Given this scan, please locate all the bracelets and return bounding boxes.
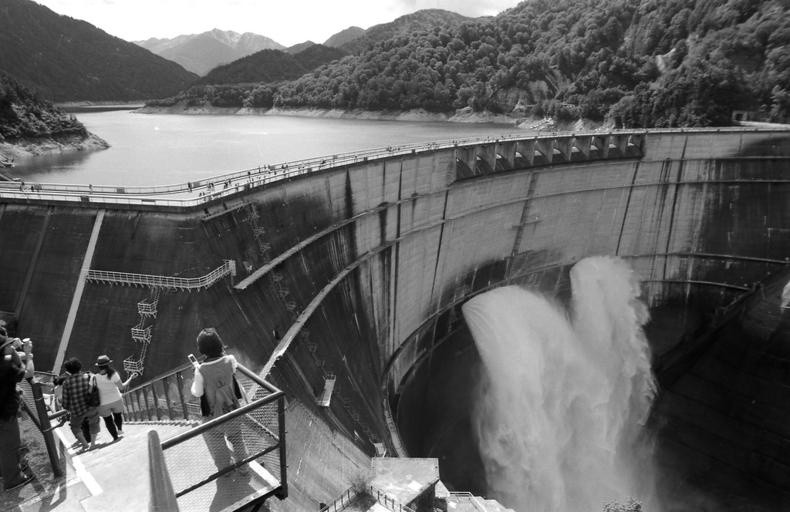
[25,353,35,359]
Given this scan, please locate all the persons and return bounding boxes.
[0,319,38,493]
[87,355,139,442]
[60,356,102,454]
[189,327,252,480]
[52,360,72,425]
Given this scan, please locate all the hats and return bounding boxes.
[94,355,114,367]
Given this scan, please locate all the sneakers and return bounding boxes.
[79,442,102,453]
[117,429,124,434]
[219,464,250,482]
[0,463,36,492]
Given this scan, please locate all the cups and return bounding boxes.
[22,338,30,344]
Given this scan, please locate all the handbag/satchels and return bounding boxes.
[88,375,100,408]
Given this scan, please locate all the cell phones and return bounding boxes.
[188,353,200,368]
